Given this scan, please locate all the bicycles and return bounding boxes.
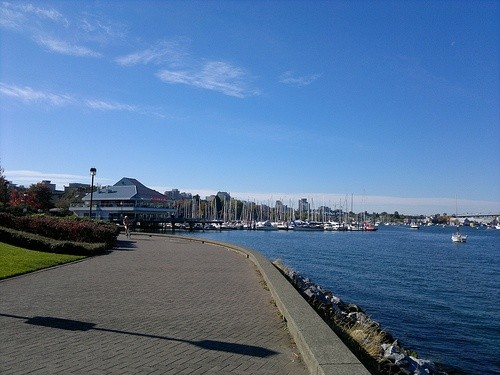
[124,225,131,240]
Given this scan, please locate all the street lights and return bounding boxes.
[90,168,96,218]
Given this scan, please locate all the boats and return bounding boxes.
[452,234,467,243]
[411,223,419,229]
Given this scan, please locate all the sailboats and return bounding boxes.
[158,192,378,232]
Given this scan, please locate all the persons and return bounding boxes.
[122,215,133,237]
